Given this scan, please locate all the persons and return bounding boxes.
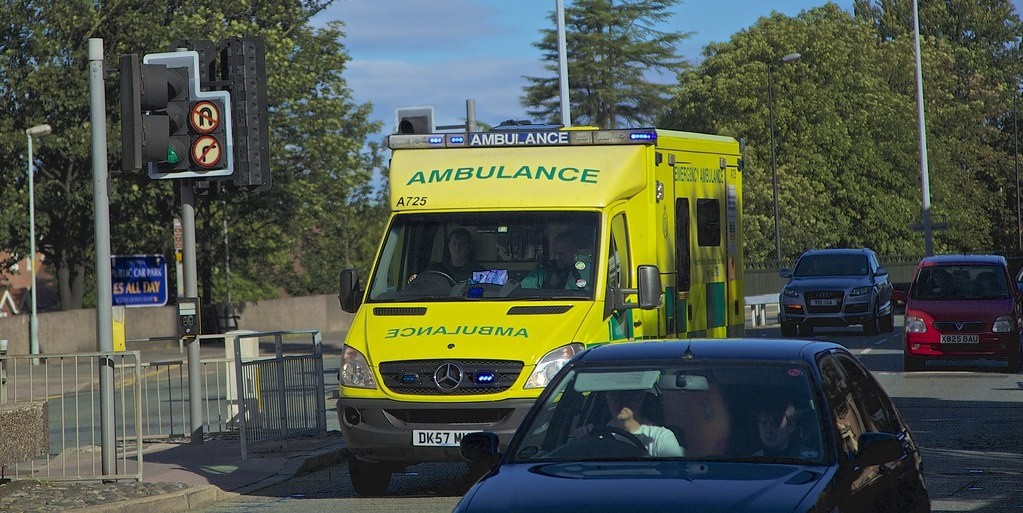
[923,268,962,295]
[407,228,487,286]
[751,400,821,458]
[977,272,1001,298]
[520,232,592,291]
[574,390,687,457]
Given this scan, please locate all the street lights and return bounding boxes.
[25,123,54,365]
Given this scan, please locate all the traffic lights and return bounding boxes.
[396,106,437,134]
[118,53,171,173]
[151,66,189,173]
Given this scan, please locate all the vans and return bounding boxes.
[335,123,748,498]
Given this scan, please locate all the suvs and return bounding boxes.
[777,246,895,338]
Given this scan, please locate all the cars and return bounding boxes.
[889,255,1023,373]
[449,335,933,513]
